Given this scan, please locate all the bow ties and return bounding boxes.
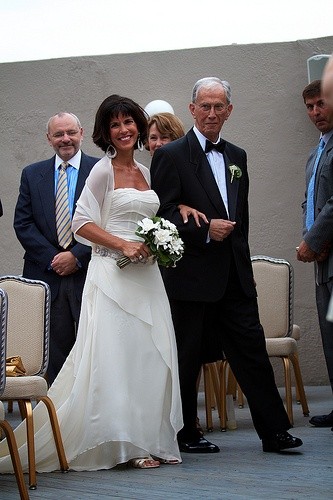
[205,139,225,153]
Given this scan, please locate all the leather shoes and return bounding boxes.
[262,430,303,452]
[176,429,221,453]
[309,410,333,427]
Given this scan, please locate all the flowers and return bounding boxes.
[229,164,243,184]
[113,216,185,270]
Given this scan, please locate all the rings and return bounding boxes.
[138,255,143,261]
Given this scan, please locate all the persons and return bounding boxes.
[296,54,333,433]
[143,109,187,159]
[12,112,104,390]
[149,76,304,455]
[0,94,187,474]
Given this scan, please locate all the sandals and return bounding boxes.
[128,453,181,468]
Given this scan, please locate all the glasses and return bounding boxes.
[191,99,229,113]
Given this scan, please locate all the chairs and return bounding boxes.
[194,254,311,432]
[0,274,70,499]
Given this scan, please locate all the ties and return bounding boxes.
[54,162,73,249]
[306,138,326,231]
